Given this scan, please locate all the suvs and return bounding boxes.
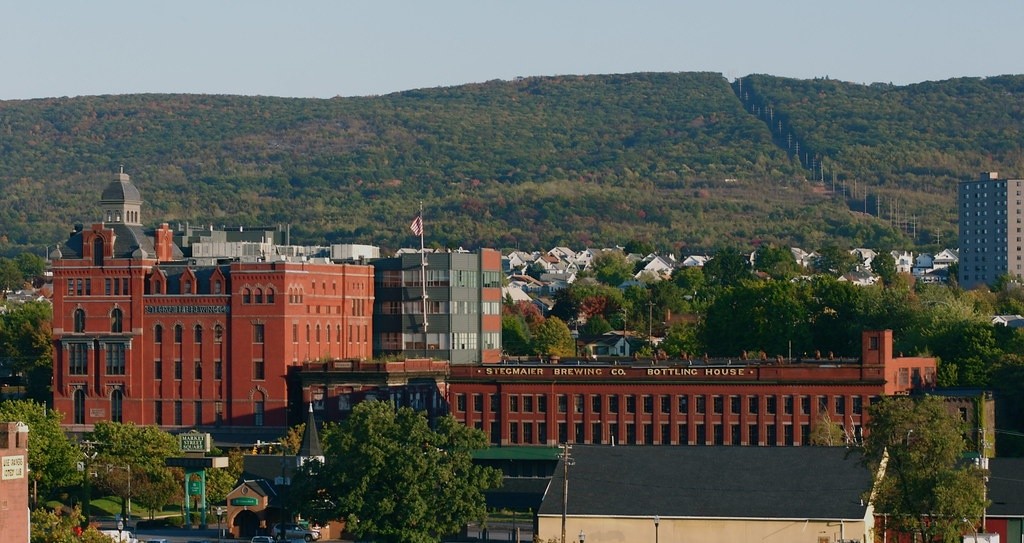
[272,522,322,542]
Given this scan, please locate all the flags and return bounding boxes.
[409,209,424,238]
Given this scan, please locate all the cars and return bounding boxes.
[251,536,275,543]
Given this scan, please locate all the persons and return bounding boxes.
[829,351,833,360]
[751,353,757,360]
[294,512,300,526]
[537,350,541,360]
[758,348,767,362]
[651,348,669,366]
[633,352,638,361]
[585,352,597,362]
[815,349,820,360]
[742,350,747,359]
[775,353,784,365]
[704,352,713,364]
[803,351,807,358]
[501,351,509,364]
[766,362,772,366]
[679,349,696,364]
